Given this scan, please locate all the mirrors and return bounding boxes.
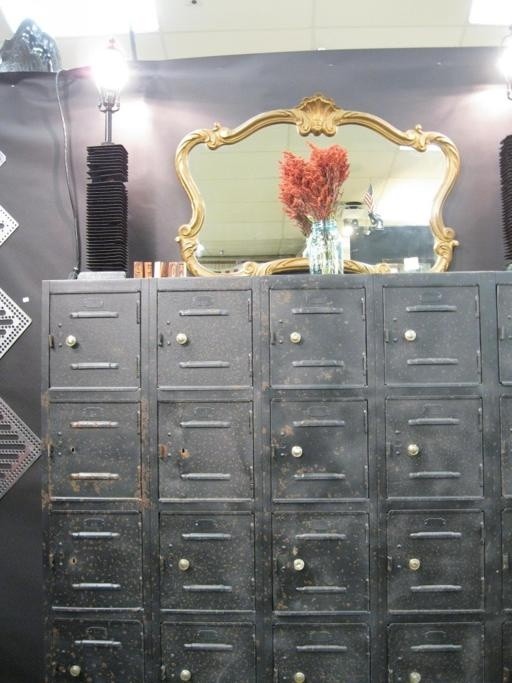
[174,93,461,278]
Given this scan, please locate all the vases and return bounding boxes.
[306,222,344,273]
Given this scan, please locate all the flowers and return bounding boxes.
[278,140,350,273]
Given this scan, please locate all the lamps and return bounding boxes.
[88,38,129,146]
[497,24,512,103]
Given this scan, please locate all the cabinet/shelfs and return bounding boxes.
[40,271,512,683]
